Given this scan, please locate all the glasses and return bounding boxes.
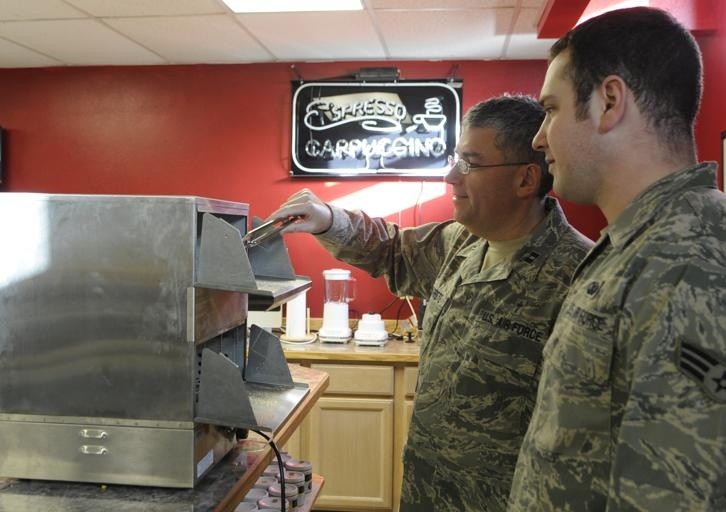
[446,155,544,174]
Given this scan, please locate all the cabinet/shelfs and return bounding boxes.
[303,363,418,509]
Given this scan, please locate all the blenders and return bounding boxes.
[318,268,358,344]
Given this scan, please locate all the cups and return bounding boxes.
[287,292,307,341]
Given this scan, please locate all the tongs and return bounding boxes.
[241,216,304,250]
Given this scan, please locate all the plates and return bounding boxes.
[279,333,318,345]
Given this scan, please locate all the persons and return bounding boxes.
[264,92,597,512]
[506,5,726,512]
